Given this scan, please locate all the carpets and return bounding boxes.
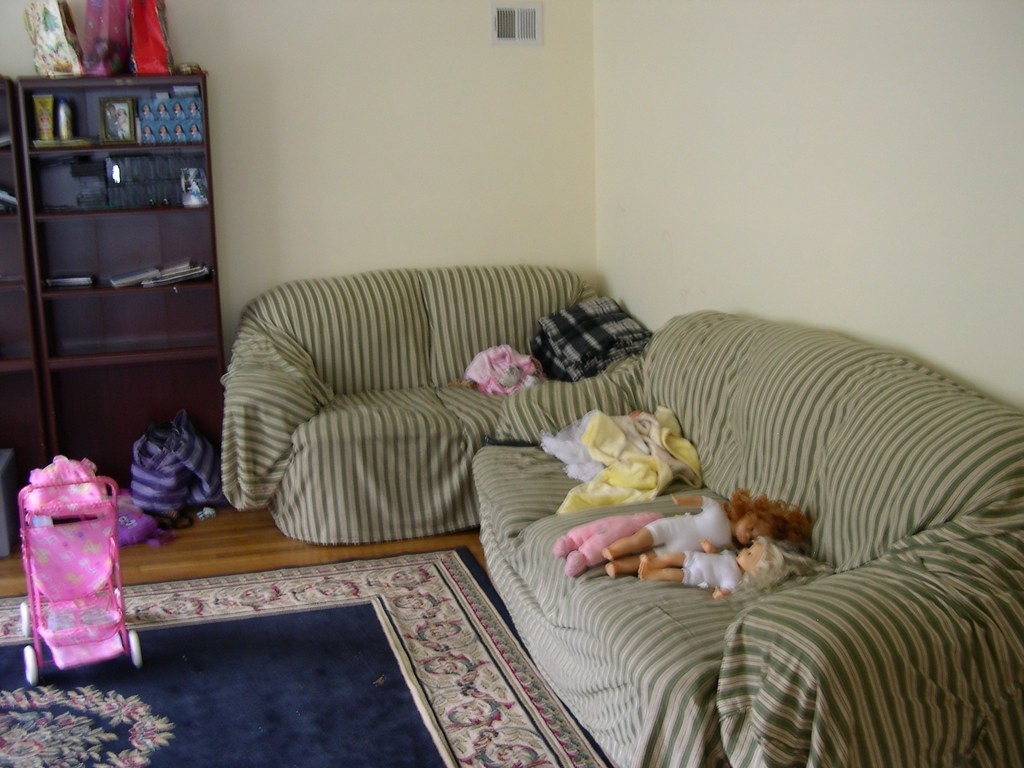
[0,545,617,768]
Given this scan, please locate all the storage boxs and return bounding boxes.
[155,153,205,181]
[69,160,110,208]
[108,183,157,208]
[155,178,183,206]
[104,154,154,184]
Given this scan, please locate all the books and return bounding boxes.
[110,256,210,288]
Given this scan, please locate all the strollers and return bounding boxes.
[17,454,144,687]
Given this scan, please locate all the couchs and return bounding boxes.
[470,310,1024,768]
[219,265,647,548]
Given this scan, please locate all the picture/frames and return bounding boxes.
[98,94,136,145]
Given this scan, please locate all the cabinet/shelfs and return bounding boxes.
[15,73,230,510]
[0,77,50,542]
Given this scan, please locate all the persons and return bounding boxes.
[554,490,819,601]
[104,101,202,196]
